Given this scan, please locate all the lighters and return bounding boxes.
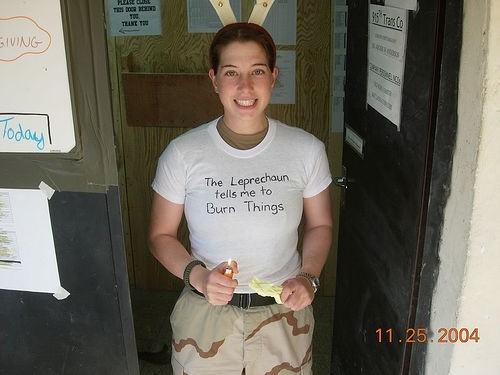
[221,257,234,281]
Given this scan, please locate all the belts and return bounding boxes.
[192,290,277,308]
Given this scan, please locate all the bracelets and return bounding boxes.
[183,259,206,290]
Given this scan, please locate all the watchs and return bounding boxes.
[295,271,321,294]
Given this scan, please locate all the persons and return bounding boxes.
[145,23,333,375]
